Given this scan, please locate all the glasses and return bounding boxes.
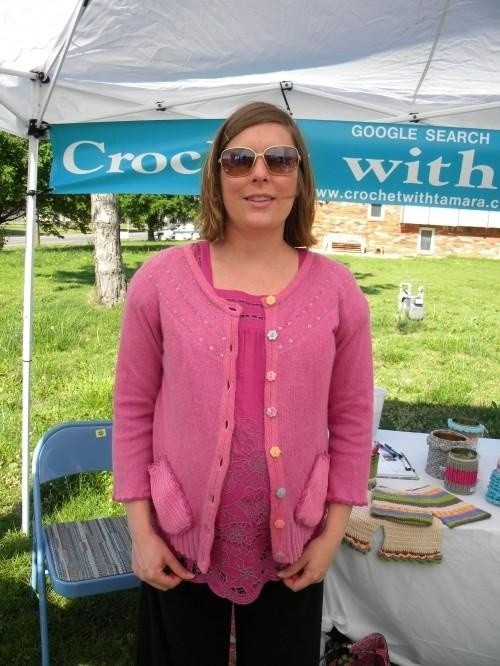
[217,146,301,176]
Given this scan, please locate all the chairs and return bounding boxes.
[30,419,141,665]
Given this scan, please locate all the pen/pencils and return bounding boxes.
[376,440,413,471]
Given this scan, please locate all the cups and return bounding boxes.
[449,418,478,453]
[427,432,466,482]
[442,450,475,495]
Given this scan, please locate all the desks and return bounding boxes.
[322,429,500,666]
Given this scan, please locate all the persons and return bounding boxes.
[112,102,374,666]
